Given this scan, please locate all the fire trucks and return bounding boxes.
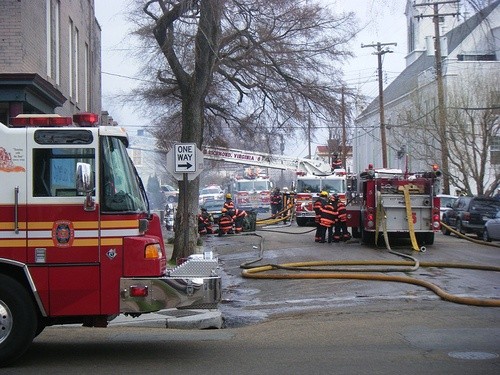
[346,165,440,247]
[123,135,356,227]
[229,168,271,213]
[1,106,223,366]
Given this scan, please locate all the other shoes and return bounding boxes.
[227,231,234,234]
[315,237,348,243]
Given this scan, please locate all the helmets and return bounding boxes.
[329,196,335,201]
[320,191,327,196]
[333,152,338,156]
[334,194,339,200]
[222,208,227,212]
[226,194,232,198]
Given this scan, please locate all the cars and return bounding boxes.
[436,195,462,221]
[439,196,500,239]
[199,185,225,206]
[160,185,180,202]
[481,210,500,241]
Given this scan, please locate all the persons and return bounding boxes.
[272,187,282,223]
[198,208,214,234]
[224,194,235,212]
[495,190,500,201]
[217,208,248,234]
[456,190,468,197]
[331,194,350,241]
[320,196,339,243]
[313,191,329,242]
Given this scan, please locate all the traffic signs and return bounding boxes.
[174,143,197,173]
